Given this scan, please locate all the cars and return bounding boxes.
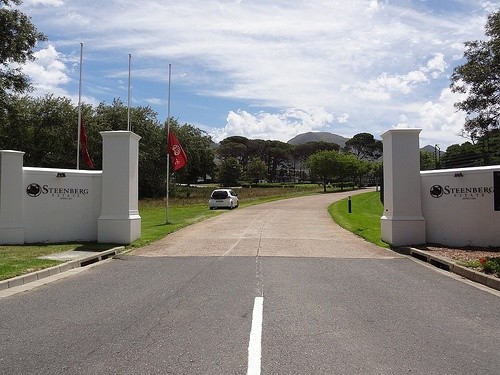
[209,189,239,209]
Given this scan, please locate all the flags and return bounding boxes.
[80,115,95,169]
[165,129,188,172]
